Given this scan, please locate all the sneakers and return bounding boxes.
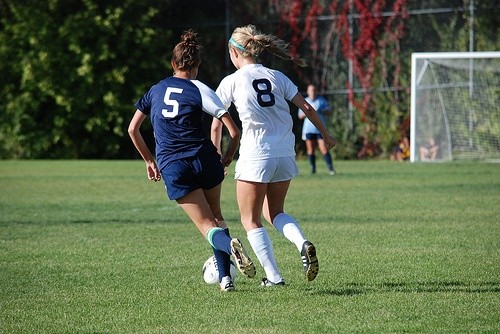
[259,277,285,287]
[229,238,256,278]
[301,241,319,281]
[219,276,235,292]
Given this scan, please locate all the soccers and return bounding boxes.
[202,255,237,284]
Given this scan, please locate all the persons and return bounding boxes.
[210,25,337,288]
[297,84,336,175]
[348,123,451,164]
[128,33,256,292]
